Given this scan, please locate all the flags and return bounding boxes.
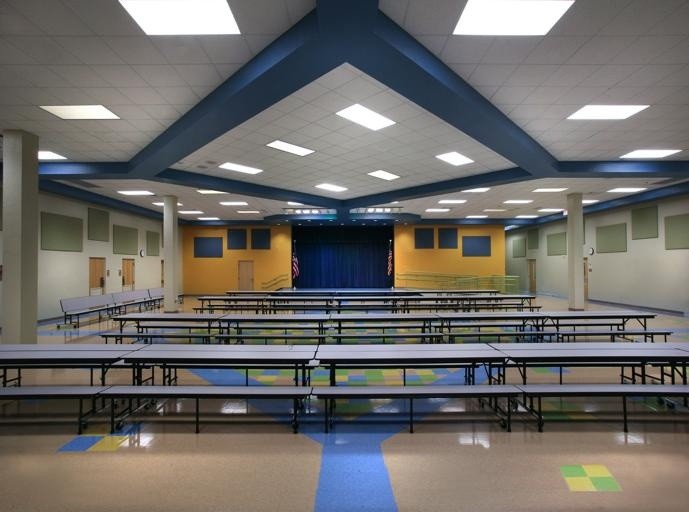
[292,241,300,280]
[386,240,393,277]
